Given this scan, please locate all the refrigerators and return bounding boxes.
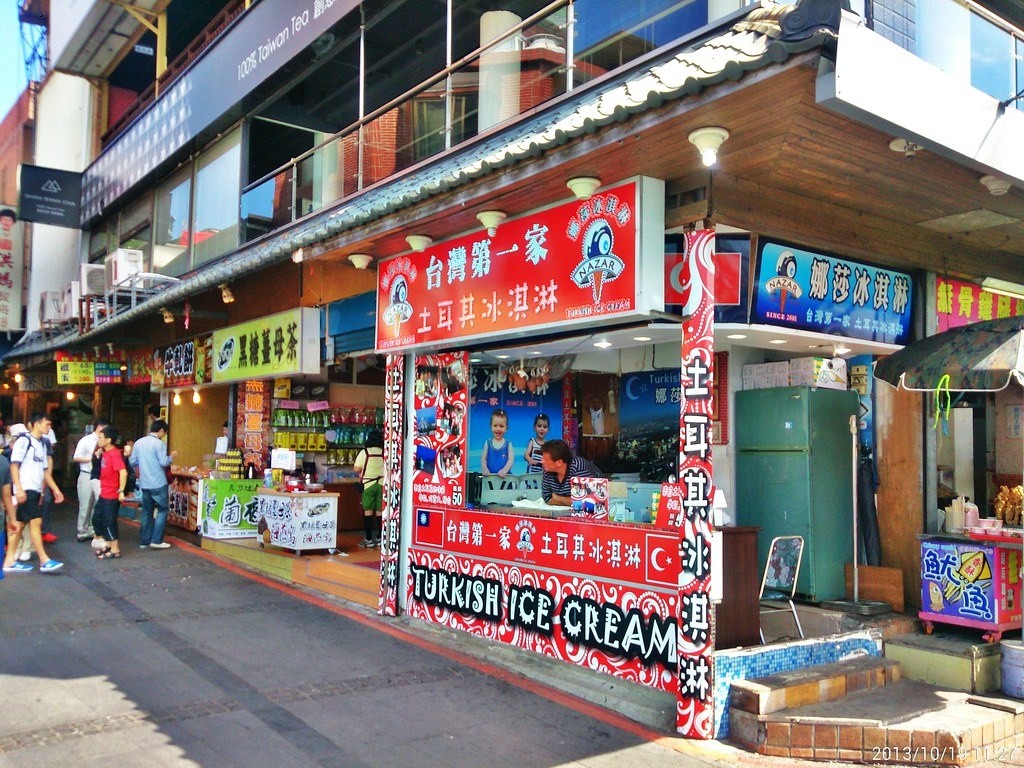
[734,388,860,602]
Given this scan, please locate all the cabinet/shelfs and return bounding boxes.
[269,421,384,476]
[323,482,364,531]
[257,488,340,555]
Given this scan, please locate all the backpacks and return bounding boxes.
[1,432,31,469]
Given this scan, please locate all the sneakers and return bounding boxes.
[375,537,381,547]
[140,544,149,549]
[14,551,33,561]
[150,541,172,549]
[358,538,375,548]
[39,559,64,573]
[1,561,35,573]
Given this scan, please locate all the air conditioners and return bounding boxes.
[40,249,144,323]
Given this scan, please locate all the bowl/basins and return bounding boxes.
[305,483,324,492]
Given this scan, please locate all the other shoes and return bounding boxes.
[90,539,106,549]
[77,529,95,542]
[41,532,58,542]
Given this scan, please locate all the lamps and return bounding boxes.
[819,344,851,356]
[160,307,174,323]
[218,284,234,303]
[80,342,115,362]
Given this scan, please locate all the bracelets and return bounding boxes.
[117,489,123,492]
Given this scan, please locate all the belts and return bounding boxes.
[80,470,92,474]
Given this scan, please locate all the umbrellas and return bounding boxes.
[871,314,1024,392]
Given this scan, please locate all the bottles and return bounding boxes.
[216,448,244,480]
[247,462,255,479]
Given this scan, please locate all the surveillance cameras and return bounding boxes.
[906,149,915,161]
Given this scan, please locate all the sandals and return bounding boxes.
[95,546,111,556]
[97,551,120,559]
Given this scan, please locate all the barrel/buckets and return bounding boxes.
[1000,639,1024,699]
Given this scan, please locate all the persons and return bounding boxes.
[353,429,384,548]
[524,413,550,489]
[129,420,178,548]
[90,425,128,560]
[0,208,17,231]
[0,412,65,572]
[73,418,110,542]
[481,408,514,479]
[540,438,605,507]
[149,404,160,422]
[222,421,228,437]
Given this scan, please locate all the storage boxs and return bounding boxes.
[742,356,848,391]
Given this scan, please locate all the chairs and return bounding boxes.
[480,472,543,506]
[760,536,804,645]
